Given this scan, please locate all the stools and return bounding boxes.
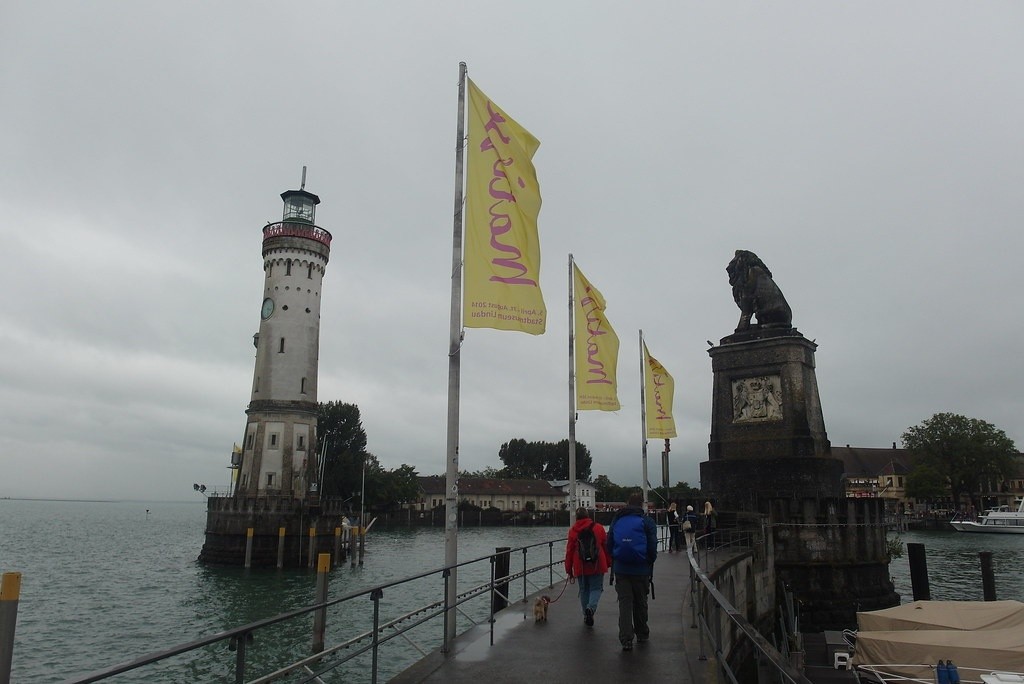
[834,653,849,669]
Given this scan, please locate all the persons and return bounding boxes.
[682,505,698,553]
[605,492,658,652]
[666,502,681,553]
[704,501,719,550]
[563,507,612,627]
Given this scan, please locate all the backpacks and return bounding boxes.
[578,522,599,564]
[610,514,655,600]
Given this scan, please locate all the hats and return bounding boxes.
[687,505,693,511]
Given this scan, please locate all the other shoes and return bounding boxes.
[669,550,672,553]
[623,640,634,650]
[637,638,649,642]
[584,607,594,626]
[676,549,681,552]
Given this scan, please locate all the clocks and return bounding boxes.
[261,298,274,319]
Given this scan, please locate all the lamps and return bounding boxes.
[194,484,200,491]
[200,485,206,492]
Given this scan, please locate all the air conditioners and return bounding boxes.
[231,452,240,465]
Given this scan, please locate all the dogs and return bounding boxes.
[533,595,551,625]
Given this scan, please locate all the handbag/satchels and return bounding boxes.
[683,514,691,530]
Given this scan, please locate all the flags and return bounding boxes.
[462,73,548,336]
[643,339,678,439]
[574,258,622,412]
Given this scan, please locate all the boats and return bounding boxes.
[949,498,1024,534]
[851,598,1024,683]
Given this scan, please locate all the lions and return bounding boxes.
[726,249,792,333]
